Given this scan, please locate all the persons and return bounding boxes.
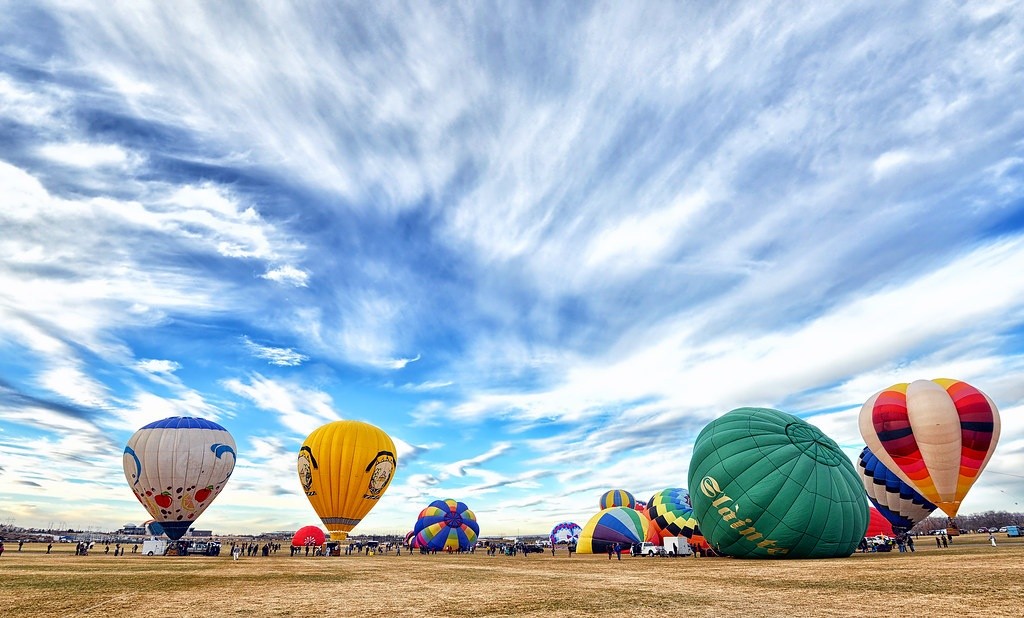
[989,533,997,547]
[673,543,678,557]
[206,544,220,556]
[936,537,942,548]
[606,542,621,560]
[75,541,84,555]
[948,533,952,543]
[941,534,948,548]
[479,540,529,557]
[916,532,918,539]
[567,543,572,558]
[290,545,332,557]
[163,542,193,556]
[690,543,703,558]
[101,540,104,545]
[46,544,52,554]
[262,541,281,556]
[0,540,4,557]
[104,546,109,554]
[114,543,124,556]
[859,534,916,553]
[419,543,477,555]
[18,540,23,551]
[345,540,414,556]
[632,542,638,557]
[105,540,110,545]
[87,540,90,549]
[132,545,138,553]
[227,541,259,560]
[336,541,341,549]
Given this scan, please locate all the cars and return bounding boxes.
[187,540,222,555]
[926,527,947,535]
[977,524,1024,538]
[315,546,324,556]
[859,534,897,549]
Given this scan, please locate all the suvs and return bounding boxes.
[522,544,545,554]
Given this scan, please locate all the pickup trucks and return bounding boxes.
[629,541,665,558]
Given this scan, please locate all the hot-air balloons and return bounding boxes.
[291,525,326,546]
[598,489,636,511]
[403,531,419,549]
[413,498,480,552]
[636,500,646,513]
[643,487,711,557]
[121,415,239,556]
[857,377,1002,537]
[297,420,398,556]
[855,446,938,545]
[548,521,583,545]
[575,507,659,554]
[687,407,871,559]
[864,505,897,539]
